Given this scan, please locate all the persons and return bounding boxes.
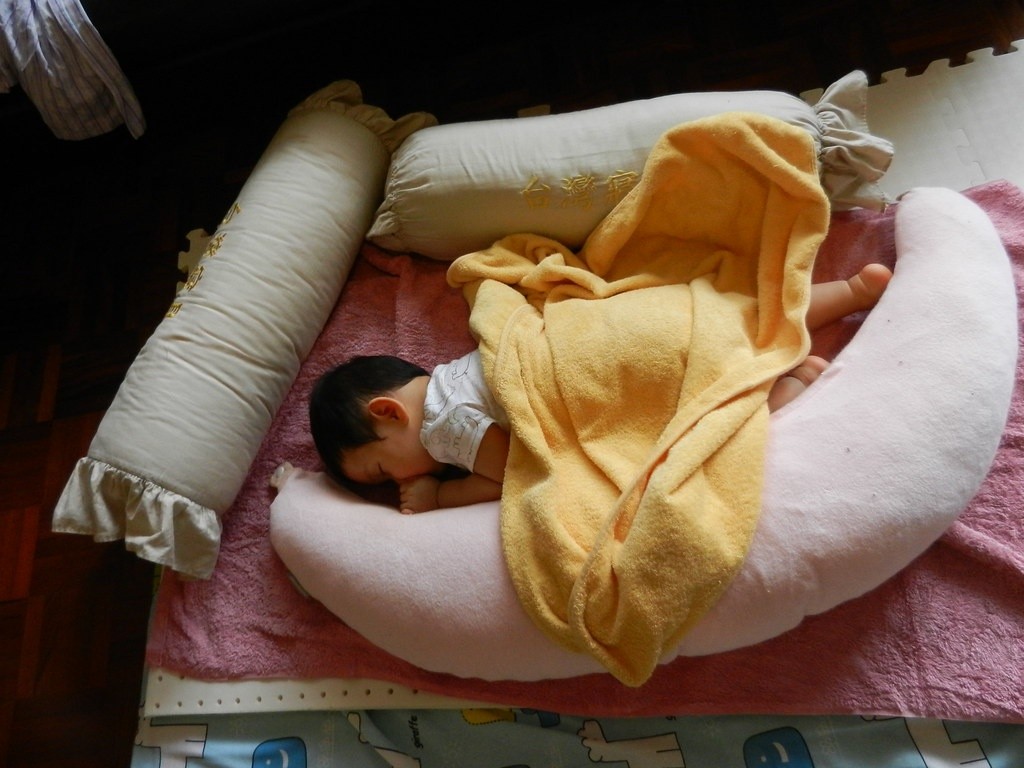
[309,263,892,513]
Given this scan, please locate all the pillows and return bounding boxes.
[46,78,438,584]
[363,68,901,257]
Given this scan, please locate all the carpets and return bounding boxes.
[143,181,1024,723]
